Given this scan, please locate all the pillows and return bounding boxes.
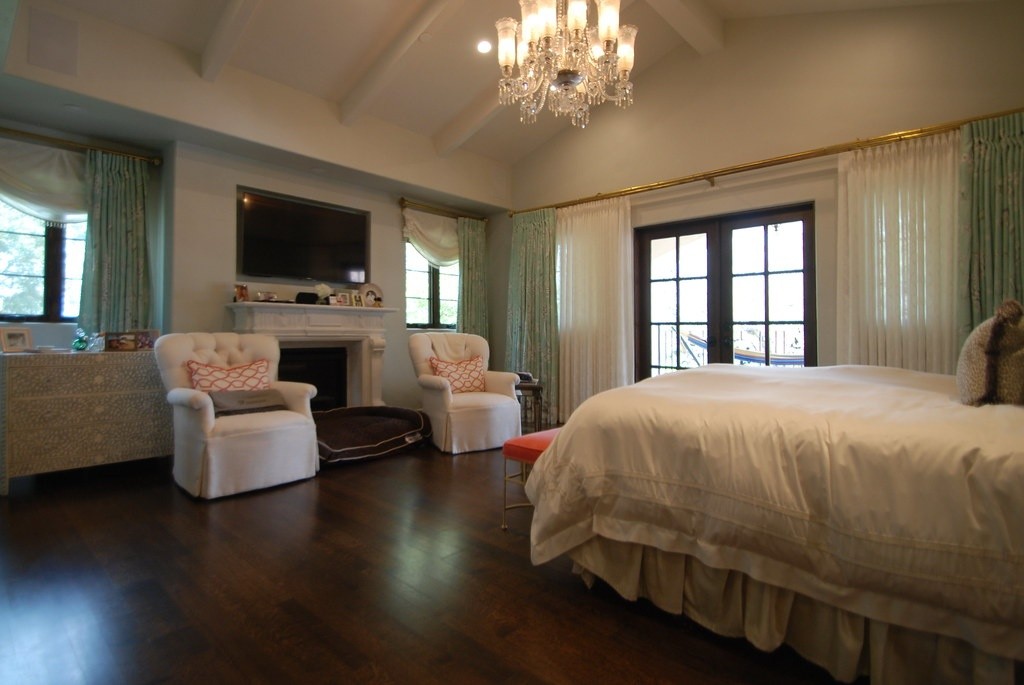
[953,298,1024,408]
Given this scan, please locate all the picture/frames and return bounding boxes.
[234,279,385,310]
[104,332,139,352]
[127,328,160,351]
[0,326,33,353]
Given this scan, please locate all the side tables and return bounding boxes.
[513,379,544,434]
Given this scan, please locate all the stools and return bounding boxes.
[500,427,565,531]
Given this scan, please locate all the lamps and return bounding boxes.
[494,0,639,130]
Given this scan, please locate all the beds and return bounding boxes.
[524,363,1024,685]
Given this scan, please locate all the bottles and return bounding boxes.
[71,329,105,351]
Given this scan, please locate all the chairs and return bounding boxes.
[409,331,524,456]
[154,331,323,500]
